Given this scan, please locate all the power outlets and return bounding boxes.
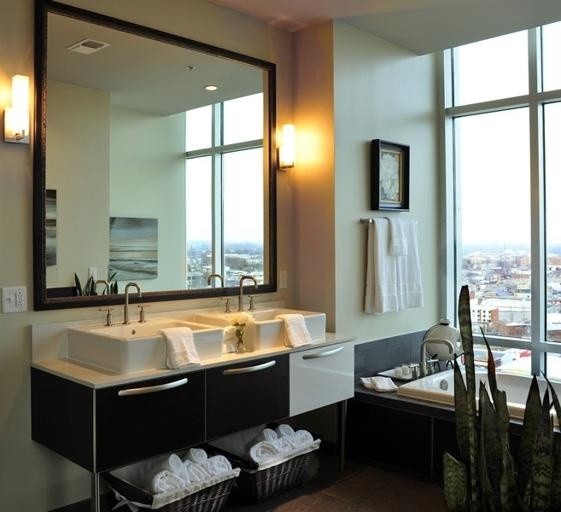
[0,286,28,314]
[88,266,98,281]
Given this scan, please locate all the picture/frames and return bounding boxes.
[370,139,409,212]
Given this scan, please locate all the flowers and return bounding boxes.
[225,313,251,349]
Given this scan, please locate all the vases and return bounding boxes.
[233,325,247,353]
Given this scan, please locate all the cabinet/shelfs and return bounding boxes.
[289,340,355,420]
[204,354,290,446]
[29,361,205,512]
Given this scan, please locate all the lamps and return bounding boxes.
[277,123,296,171]
[0,73,31,145]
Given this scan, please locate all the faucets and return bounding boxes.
[125,282,142,304]
[93,280,110,296]
[240,276,258,297]
[421,338,455,363]
[208,275,224,289]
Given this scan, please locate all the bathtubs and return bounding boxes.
[399,368,561,427]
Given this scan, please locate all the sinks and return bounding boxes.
[195,308,326,352]
[69,319,224,371]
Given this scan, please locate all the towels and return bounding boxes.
[364,216,423,315]
[158,327,202,371]
[360,376,399,393]
[232,424,314,464]
[135,447,232,493]
[274,313,313,348]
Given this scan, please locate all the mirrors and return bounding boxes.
[32,0,276,311]
